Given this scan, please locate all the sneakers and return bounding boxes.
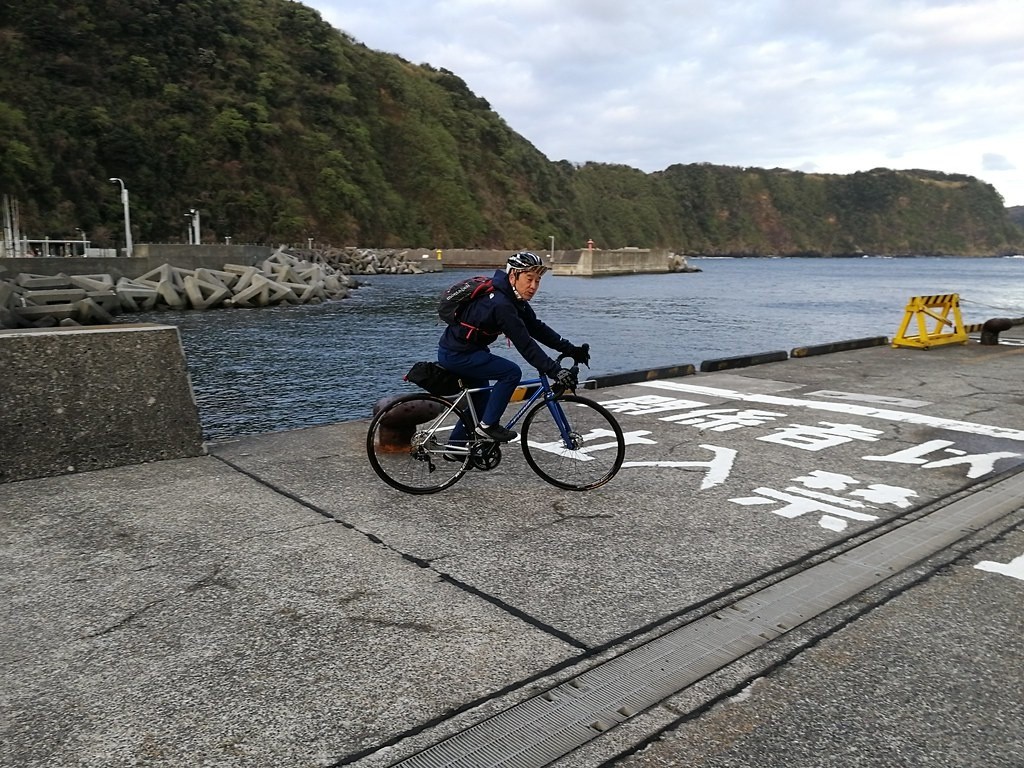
[442,420,518,464]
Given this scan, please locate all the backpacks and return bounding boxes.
[438,276,497,327]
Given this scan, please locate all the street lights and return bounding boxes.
[549,235,554,259]
[183,213,197,228]
[189,209,200,245]
[109,177,133,258]
[74,227,86,255]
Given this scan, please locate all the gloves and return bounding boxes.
[547,366,579,390]
[563,341,591,364]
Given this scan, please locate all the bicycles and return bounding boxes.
[367,342,625,495]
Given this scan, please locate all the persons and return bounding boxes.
[437,251,591,464]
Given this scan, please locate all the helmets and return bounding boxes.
[505,251,548,275]
